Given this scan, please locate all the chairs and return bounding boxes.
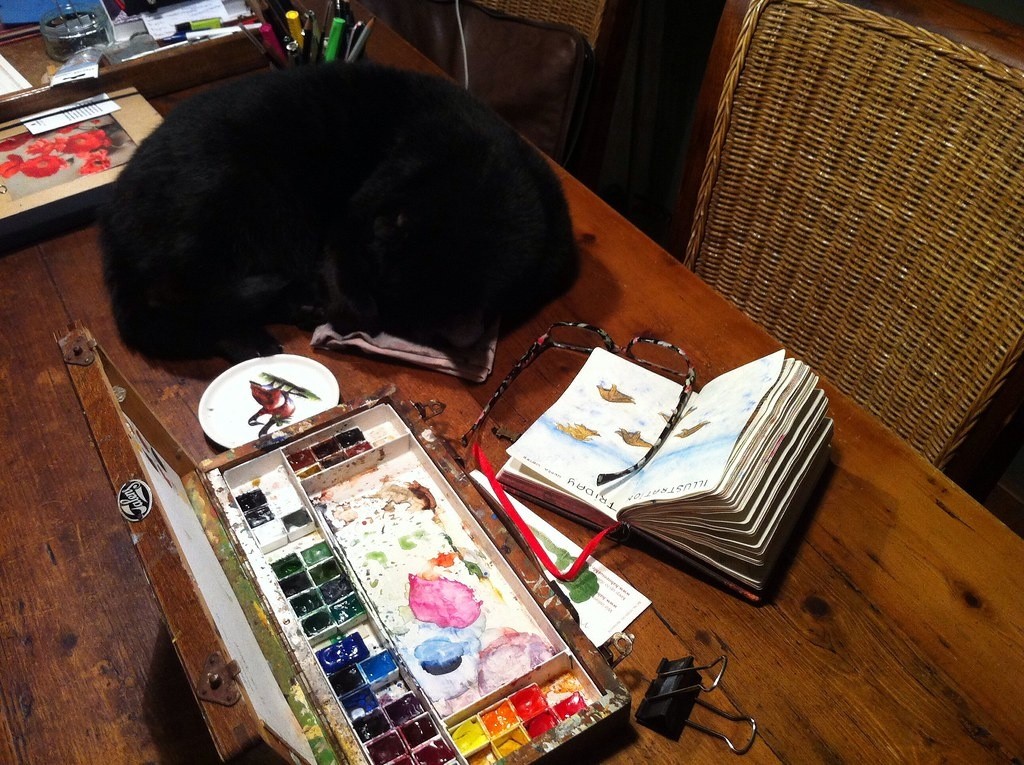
[355,0,644,190]
[661,0,1024,486]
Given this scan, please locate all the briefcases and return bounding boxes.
[56,319,632,765]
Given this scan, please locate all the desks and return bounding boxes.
[0,0,1024,765]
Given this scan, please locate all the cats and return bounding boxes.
[99,62,576,365]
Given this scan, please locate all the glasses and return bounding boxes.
[460,321,696,486]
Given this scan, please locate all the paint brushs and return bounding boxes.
[239,0,355,53]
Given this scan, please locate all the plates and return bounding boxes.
[198,354,341,450]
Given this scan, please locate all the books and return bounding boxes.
[495,349,835,604]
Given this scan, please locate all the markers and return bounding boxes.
[259,23,286,60]
[322,17,346,61]
[286,10,305,48]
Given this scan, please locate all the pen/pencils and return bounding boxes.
[304,15,313,54]
[349,17,375,63]
